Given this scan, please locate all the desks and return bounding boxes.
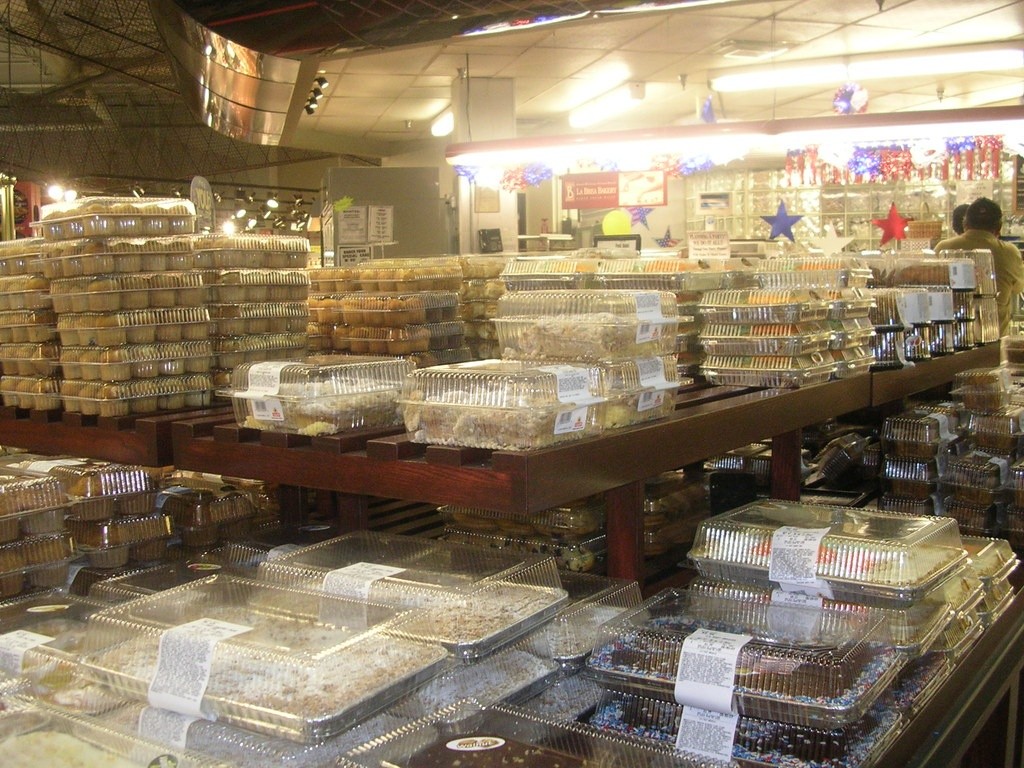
[170,370,869,586]
[2,408,233,470]
[871,341,1001,410]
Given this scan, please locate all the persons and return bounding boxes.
[952,204,970,235]
[933,196,1024,337]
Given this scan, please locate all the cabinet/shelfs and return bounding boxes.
[687,165,1002,249]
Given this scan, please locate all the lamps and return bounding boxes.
[566,74,647,128]
[426,112,453,141]
[133,180,308,234]
[304,77,330,115]
[707,36,1023,97]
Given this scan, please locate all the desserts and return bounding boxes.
[0,205,1024,768]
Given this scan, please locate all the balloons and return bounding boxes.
[833,84,868,114]
[602,209,631,235]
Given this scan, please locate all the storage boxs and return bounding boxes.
[1,199,1024,767]
